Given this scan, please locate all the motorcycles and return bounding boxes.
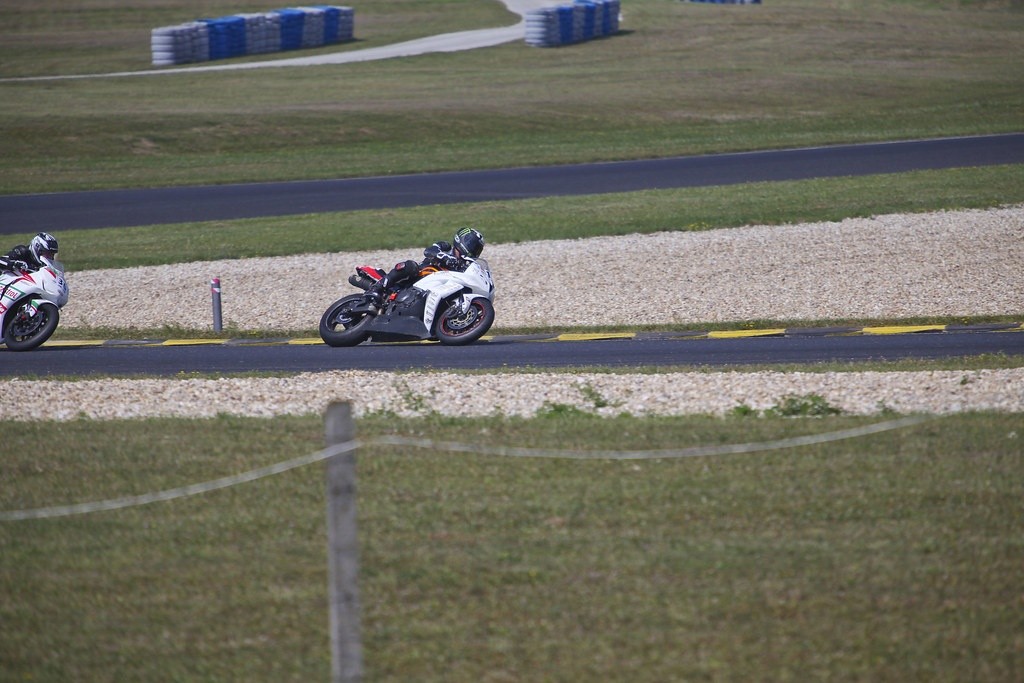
[318,252,495,347]
[0,252,70,351]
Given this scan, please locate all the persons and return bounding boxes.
[0,232,59,272]
[363,227,485,298]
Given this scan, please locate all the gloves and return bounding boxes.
[8,259,28,272]
[442,255,461,270]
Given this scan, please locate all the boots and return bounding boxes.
[363,275,393,300]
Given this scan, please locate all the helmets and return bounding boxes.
[453,227,484,259]
[29,231,59,266]
